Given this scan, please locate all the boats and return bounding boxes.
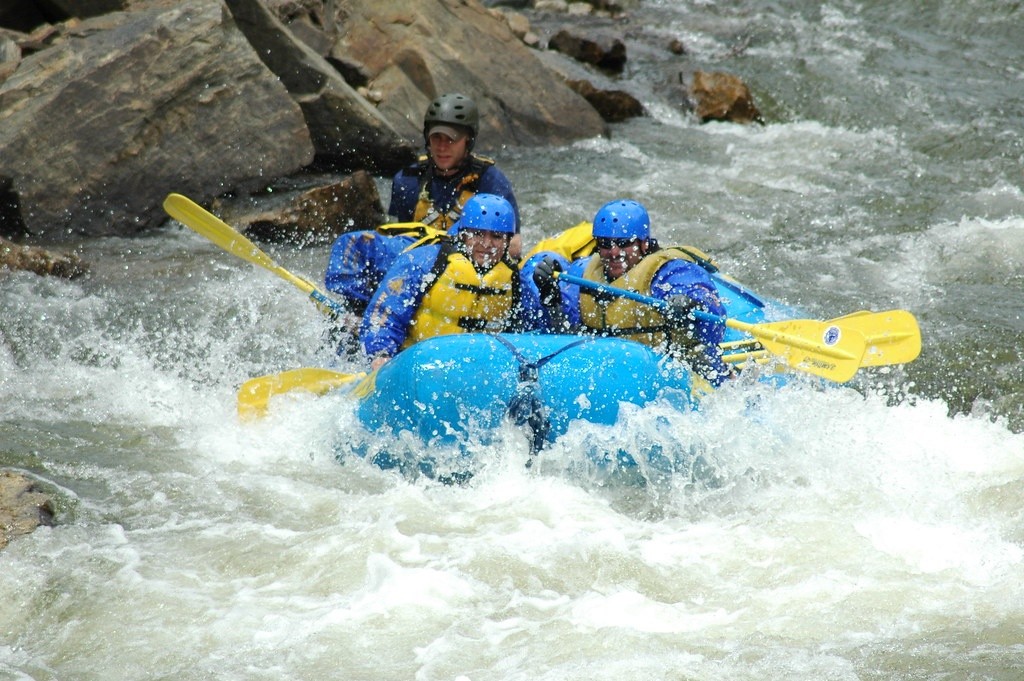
[321,220,855,489]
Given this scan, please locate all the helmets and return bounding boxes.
[425,92,479,148]
[592,199,650,240]
[458,194,516,235]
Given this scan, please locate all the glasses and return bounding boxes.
[594,236,636,249]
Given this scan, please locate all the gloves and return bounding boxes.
[533,257,563,307]
[661,294,705,330]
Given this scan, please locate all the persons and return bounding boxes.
[325,91,522,359]
[360,194,728,389]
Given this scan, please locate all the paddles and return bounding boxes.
[718,309,923,368]
[238,366,375,419]
[552,271,865,384]
[160,190,347,319]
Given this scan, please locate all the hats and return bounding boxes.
[427,123,469,143]
